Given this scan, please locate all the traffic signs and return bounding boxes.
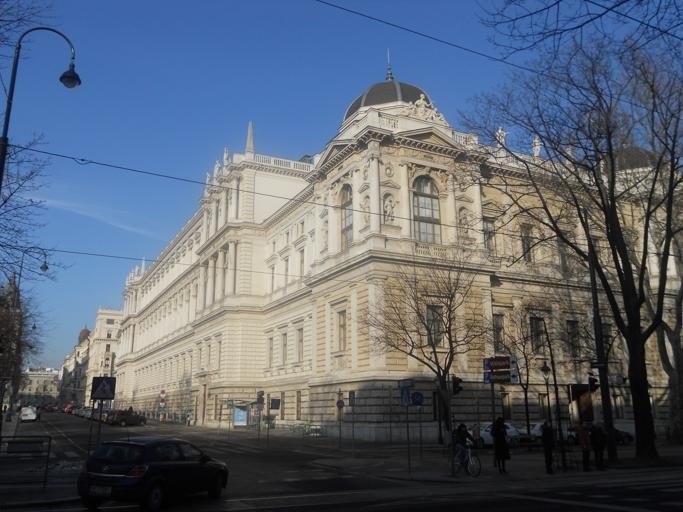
[483,356,516,370]
[484,371,517,384]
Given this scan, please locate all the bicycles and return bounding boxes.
[447,439,481,476]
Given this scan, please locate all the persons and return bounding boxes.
[543,422,555,474]
[580,426,593,472]
[454,424,471,464]
[491,417,511,474]
[591,426,608,468]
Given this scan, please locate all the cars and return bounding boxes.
[61,404,145,427]
[76,436,230,508]
[516,422,576,446]
[19,407,37,422]
[465,421,519,448]
[572,424,633,445]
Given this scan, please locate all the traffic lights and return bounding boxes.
[452,376,462,394]
[588,377,599,392]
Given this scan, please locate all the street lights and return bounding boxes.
[0,26,81,189]
[540,361,552,427]
[2,247,48,404]
